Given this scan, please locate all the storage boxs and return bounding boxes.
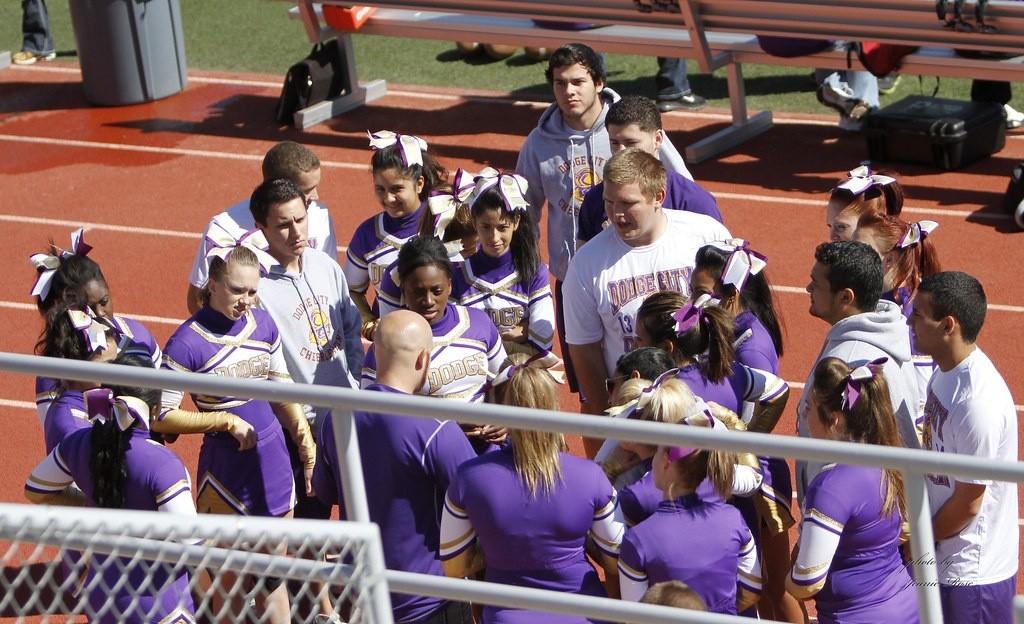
[865,95,1007,167]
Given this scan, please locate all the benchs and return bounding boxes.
[289,1,1024,162]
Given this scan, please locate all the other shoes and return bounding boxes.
[1003,104,1024,128]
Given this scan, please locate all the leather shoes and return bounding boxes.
[656,93,706,113]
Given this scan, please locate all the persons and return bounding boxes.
[24,5,1023,624]
[792,239,933,516]
[906,272,1020,624]
[11,0,57,66]
[560,146,731,452]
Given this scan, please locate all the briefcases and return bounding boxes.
[862,94,1009,173]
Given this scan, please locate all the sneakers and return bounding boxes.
[877,74,902,94]
[11,50,56,65]
[816,81,868,121]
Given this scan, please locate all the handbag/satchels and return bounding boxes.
[274,39,346,121]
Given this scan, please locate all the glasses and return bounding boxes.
[605,373,631,394]
